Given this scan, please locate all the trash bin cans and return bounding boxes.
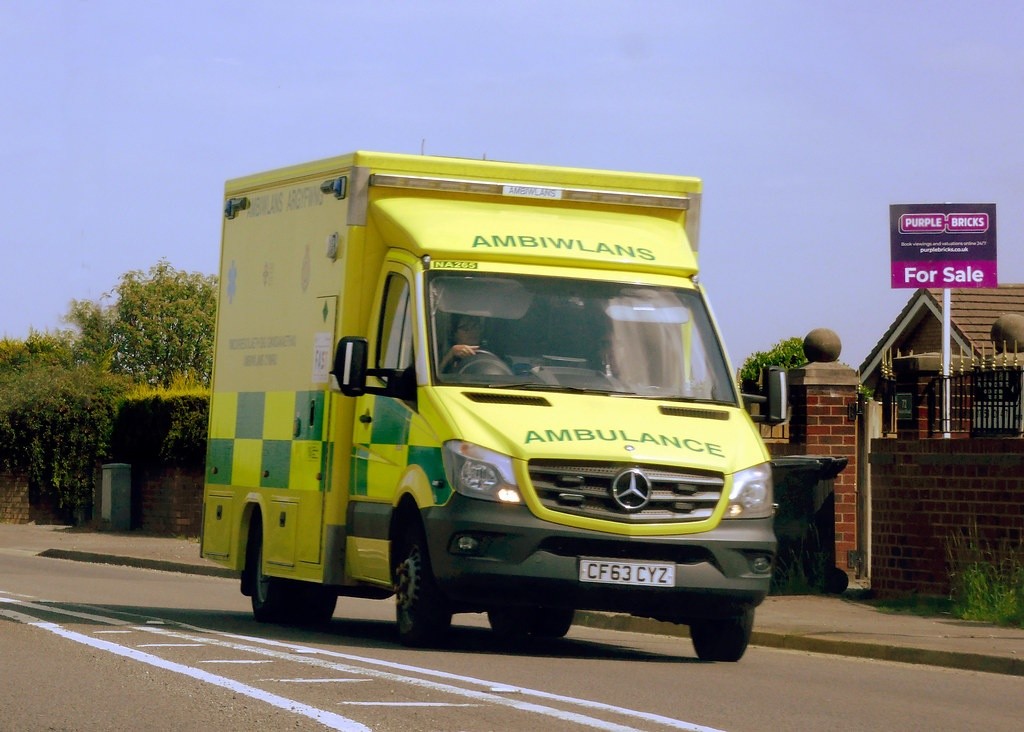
[764,455,850,597]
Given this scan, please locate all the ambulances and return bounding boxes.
[201,150,787,663]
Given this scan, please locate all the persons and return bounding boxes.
[439,314,513,374]
[594,332,657,387]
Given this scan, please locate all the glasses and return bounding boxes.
[457,322,483,332]
